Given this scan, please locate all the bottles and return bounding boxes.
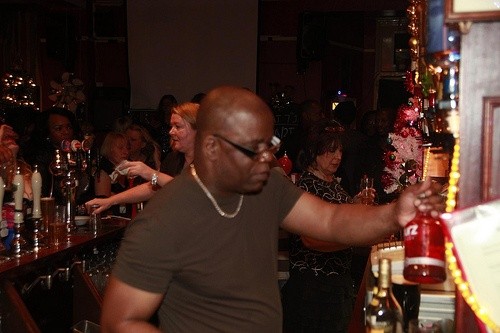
[13,210,24,227]
[363,258,404,333]
[31,163,43,219]
[411,88,452,184]
[64,170,77,225]
[12,165,26,211]
[402,182,446,285]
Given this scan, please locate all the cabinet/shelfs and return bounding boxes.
[372,16,410,110]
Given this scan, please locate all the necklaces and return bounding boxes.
[308,165,332,182]
[190,163,244,218]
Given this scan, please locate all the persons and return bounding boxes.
[332,100,394,199]
[286,99,325,172]
[29,109,84,198]
[100,85,447,332]
[124,124,160,163]
[151,94,178,151]
[84,102,199,214]
[95,131,134,219]
[286,131,376,333]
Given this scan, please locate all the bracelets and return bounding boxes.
[152,171,160,184]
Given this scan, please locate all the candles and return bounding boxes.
[14,167,25,223]
[0,177,6,221]
[31,165,43,220]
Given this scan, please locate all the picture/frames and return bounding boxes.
[443,0,500,27]
[478,95,500,202]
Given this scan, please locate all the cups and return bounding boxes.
[360,175,374,205]
[89,204,100,232]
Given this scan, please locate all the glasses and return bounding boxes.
[213,133,281,163]
